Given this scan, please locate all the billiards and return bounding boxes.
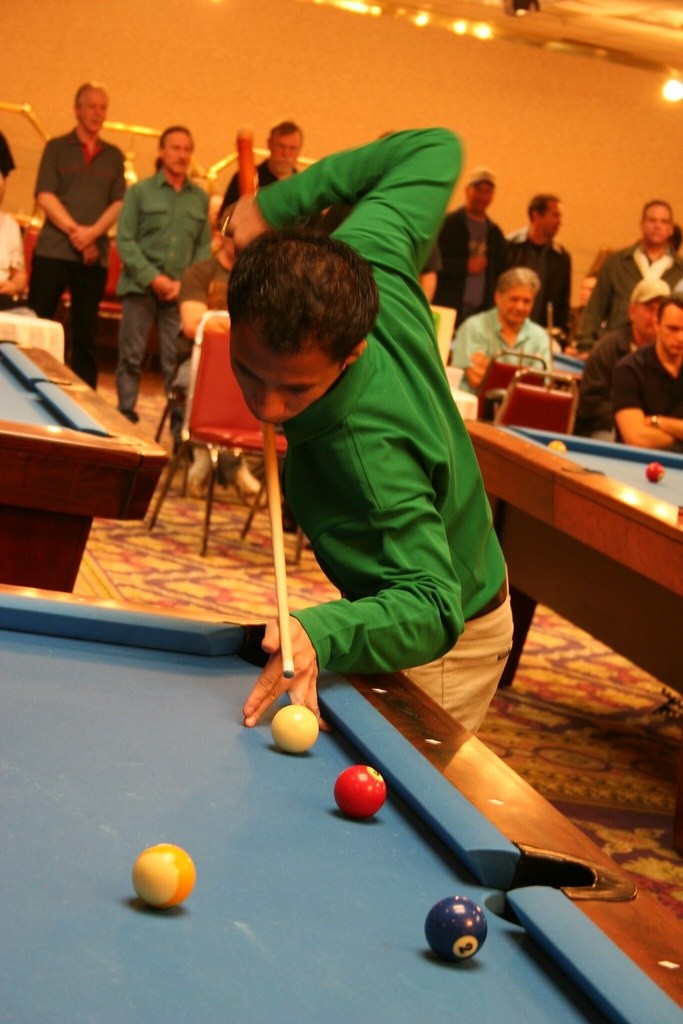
[548,441,566,453]
[646,463,665,482]
[269,705,319,754]
[334,764,388,818]
[132,843,196,907]
[424,896,489,961]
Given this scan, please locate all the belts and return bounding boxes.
[466,578,508,622]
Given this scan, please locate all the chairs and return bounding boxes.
[12,216,44,301]
[145,310,304,569]
[61,230,133,323]
[478,350,549,412]
[490,366,581,434]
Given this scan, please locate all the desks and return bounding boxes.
[462,416,683,693]
[0,578,683,1024]
[0,339,172,595]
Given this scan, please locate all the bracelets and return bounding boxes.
[651,414,657,425]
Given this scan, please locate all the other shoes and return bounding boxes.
[121,410,139,423]
[187,460,213,499]
[221,467,268,509]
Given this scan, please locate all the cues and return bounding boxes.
[547,303,554,388]
[237,125,295,678]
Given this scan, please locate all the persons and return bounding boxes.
[577,276,671,442]
[431,167,509,330]
[0,132,28,312]
[610,292,683,452]
[27,80,127,391]
[576,200,683,360]
[116,126,212,420]
[226,126,514,738]
[504,193,572,353]
[217,121,304,236]
[450,268,551,396]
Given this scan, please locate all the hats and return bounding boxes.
[629,276,672,303]
[466,170,496,191]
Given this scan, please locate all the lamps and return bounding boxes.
[504,0,542,16]
